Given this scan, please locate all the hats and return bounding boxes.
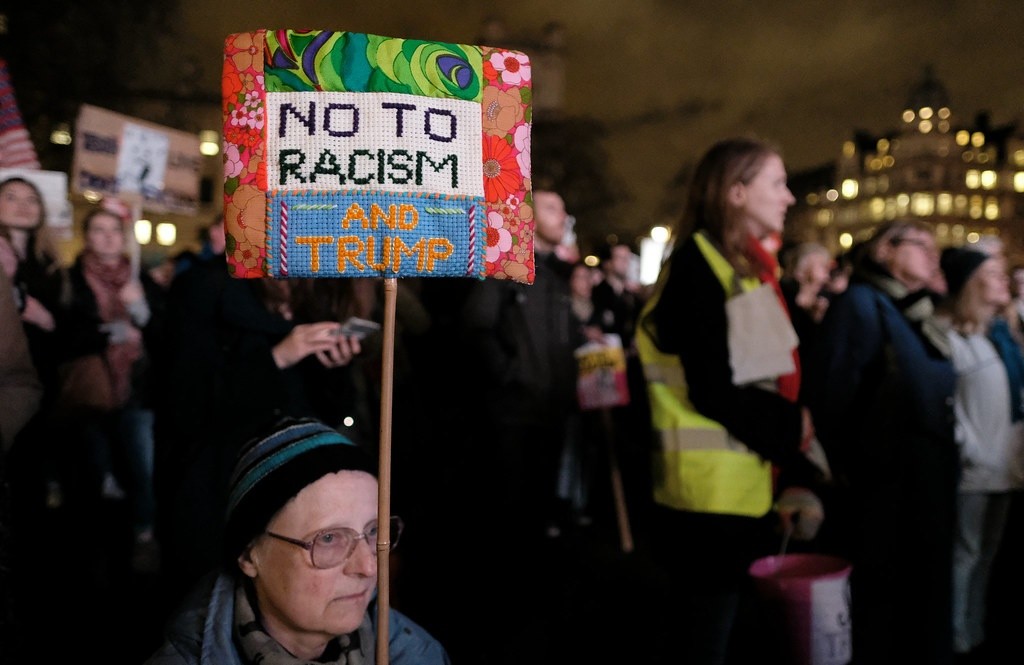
[226,414,377,558]
[940,246,987,295]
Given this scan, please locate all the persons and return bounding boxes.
[632,133,833,665]
[0,177,1024,665]
[145,415,449,665]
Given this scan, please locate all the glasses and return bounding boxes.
[263,515,405,567]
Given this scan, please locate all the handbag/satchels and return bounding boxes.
[576,334,629,409]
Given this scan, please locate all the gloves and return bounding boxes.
[774,487,824,540]
